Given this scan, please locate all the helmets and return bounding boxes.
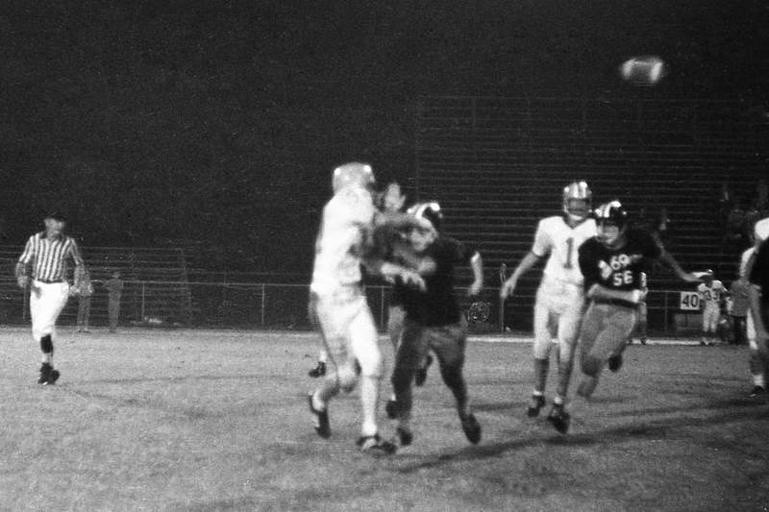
[400,202,444,237]
[561,180,592,221]
[330,161,375,194]
[593,200,628,235]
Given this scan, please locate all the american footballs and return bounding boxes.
[621,55,664,87]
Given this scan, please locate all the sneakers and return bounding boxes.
[386,401,399,418]
[307,361,326,378]
[416,354,434,385]
[457,406,482,445]
[747,383,768,397]
[307,389,332,438]
[577,374,600,397]
[36,362,59,385]
[546,398,567,426]
[395,421,412,446]
[355,432,398,458]
[527,394,546,418]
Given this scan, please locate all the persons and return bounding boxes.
[75,272,93,333]
[16,210,86,387]
[102,268,124,333]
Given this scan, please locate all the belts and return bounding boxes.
[30,276,64,283]
